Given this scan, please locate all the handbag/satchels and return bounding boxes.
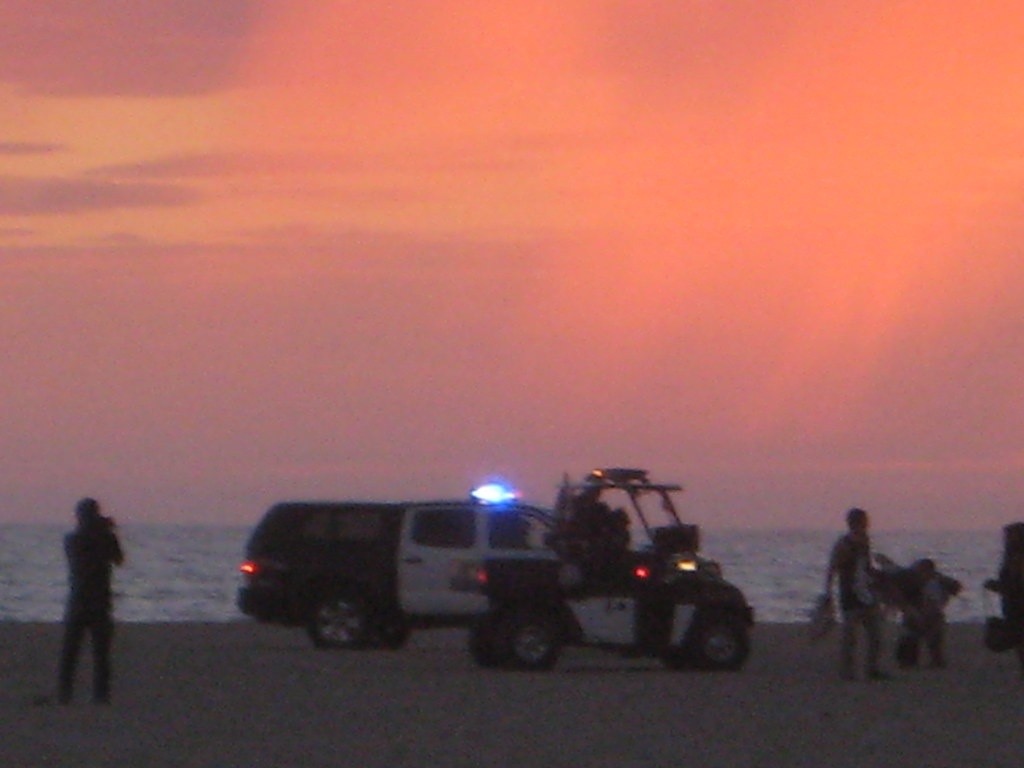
[985,616,1014,653]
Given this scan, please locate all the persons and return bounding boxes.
[885,558,949,672]
[56,497,125,706]
[983,521,1024,665]
[826,509,887,687]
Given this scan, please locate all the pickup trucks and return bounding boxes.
[234,481,647,653]
